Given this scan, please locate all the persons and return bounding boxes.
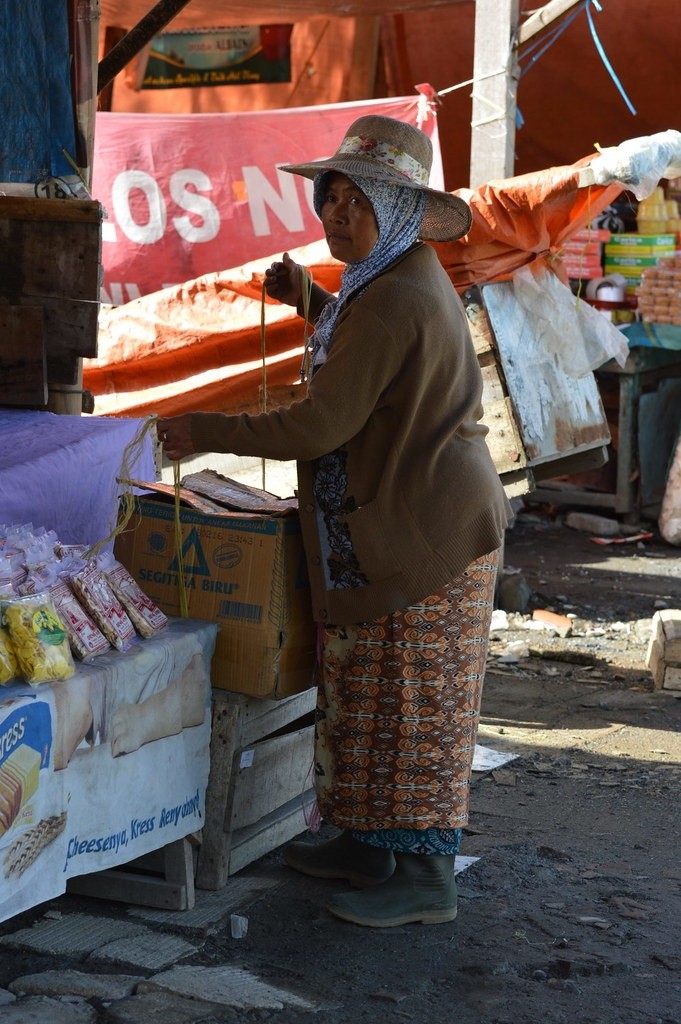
[154,115,516,929]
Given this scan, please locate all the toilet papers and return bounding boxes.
[584,272,627,325]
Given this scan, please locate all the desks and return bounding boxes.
[0,620,220,909]
[525,350,681,513]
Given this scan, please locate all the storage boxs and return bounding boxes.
[113,469,317,701]
[561,230,675,297]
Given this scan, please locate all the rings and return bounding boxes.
[163,432,167,440]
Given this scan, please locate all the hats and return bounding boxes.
[278,115,473,241]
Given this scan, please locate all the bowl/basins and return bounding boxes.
[636,189,681,251]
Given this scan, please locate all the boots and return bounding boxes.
[281,829,394,885]
[328,847,458,925]
